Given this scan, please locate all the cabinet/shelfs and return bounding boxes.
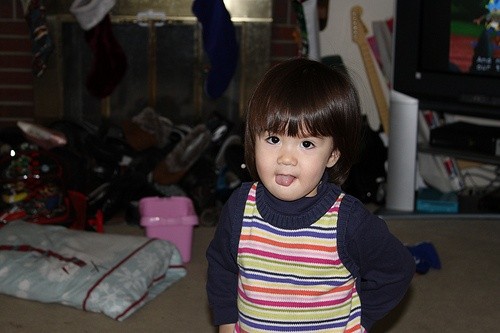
[372,209,500,333]
[32,14,276,126]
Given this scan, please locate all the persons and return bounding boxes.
[205,59,416,332]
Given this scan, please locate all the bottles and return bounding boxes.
[2,149,32,226]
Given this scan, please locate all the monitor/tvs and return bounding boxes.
[392,0,500,119]
